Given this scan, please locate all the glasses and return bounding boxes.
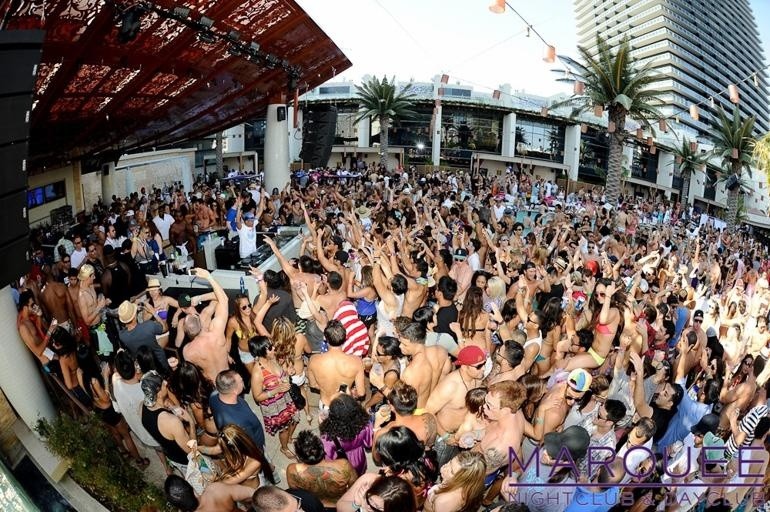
[640,460,647,474]
[376,348,387,356]
[240,303,252,311]
[693,303,719,323]
[290,493,302,512]
[36,238,97,272]
[564,392,581,402]
[365,492,384,512]
[525,292,605,349]
[266,343,275,352]
[328,141,768,272]
[597,405,612,425]
[706,360,752,370]
[468,360,495,414]
[646,271,658,294]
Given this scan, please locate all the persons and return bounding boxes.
[9,156,770,511]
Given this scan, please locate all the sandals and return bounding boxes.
[279,436,298,459]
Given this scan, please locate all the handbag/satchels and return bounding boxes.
[286,371,306,410]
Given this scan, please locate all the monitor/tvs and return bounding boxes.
[27,186,44,209]
[44,179,66,204]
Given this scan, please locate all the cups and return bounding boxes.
[314,276,322,285]
[459,432,474,448]
[574,296,586,311]
[33,304,41,315]
[279,376,290,384]
[99,294,105,304]
[561,297,569,311]
[379,403,391,423]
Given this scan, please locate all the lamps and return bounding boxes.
[113,3,301,92]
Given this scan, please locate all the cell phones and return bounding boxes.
[188,269,199,277]
[339,384,347,392]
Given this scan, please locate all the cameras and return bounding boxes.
[138,301,144,306]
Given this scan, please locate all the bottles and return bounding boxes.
[239,277,246,296]
[320,339,328,354]
[169,250,175,259]
[164,400,183,416]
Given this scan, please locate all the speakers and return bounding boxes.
[103,164,109,175]
[277,106,285,121]
[298,104,338,168]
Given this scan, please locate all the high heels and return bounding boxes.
[306,414,313,425]
[124,452,151,471]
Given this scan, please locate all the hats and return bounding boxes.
[453,247,467,258]
[551,257,566,271]
[76,263,95,281]
[694,309,716,337]
[543,425,590,460]
[125,209,135,217]
[566,367,594,392]
[245,213,257,221]
[690,413,729,467]
[118,277,164,324]
[139,369,163,408]
[178,293,191,308]
[450,345,486,365]
